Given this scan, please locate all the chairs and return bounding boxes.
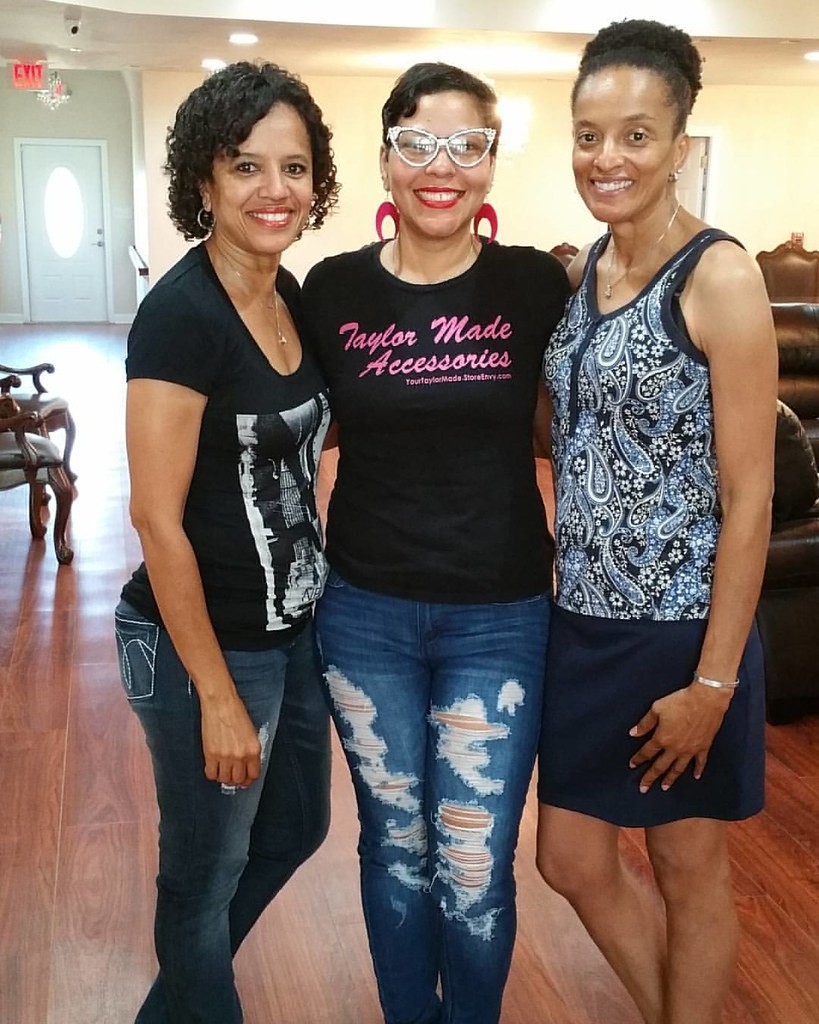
[0,361,79,566]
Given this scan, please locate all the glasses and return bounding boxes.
[386,125,498,169]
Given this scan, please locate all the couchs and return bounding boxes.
[534,240,819,728]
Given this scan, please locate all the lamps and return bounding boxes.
[36,71,72,111]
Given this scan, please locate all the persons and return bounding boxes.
[115,61,333,1024]
[536,19,780,1024]
[300,62,573,1024]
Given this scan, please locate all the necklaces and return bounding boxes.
[209,235,289,344]
[604,203,681,297]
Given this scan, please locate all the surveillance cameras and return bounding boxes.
[64,20,81,37]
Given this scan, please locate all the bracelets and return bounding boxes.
[693,671,740,689]
